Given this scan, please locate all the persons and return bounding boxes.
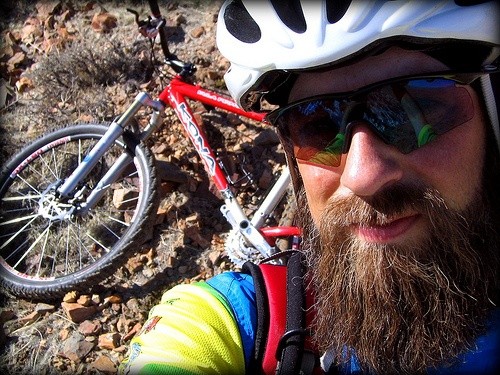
[117,0,500,375]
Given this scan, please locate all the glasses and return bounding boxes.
[265,61,498,168]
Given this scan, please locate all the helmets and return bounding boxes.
[215,0,500,112]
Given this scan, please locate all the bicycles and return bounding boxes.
[0,0,307,301]
